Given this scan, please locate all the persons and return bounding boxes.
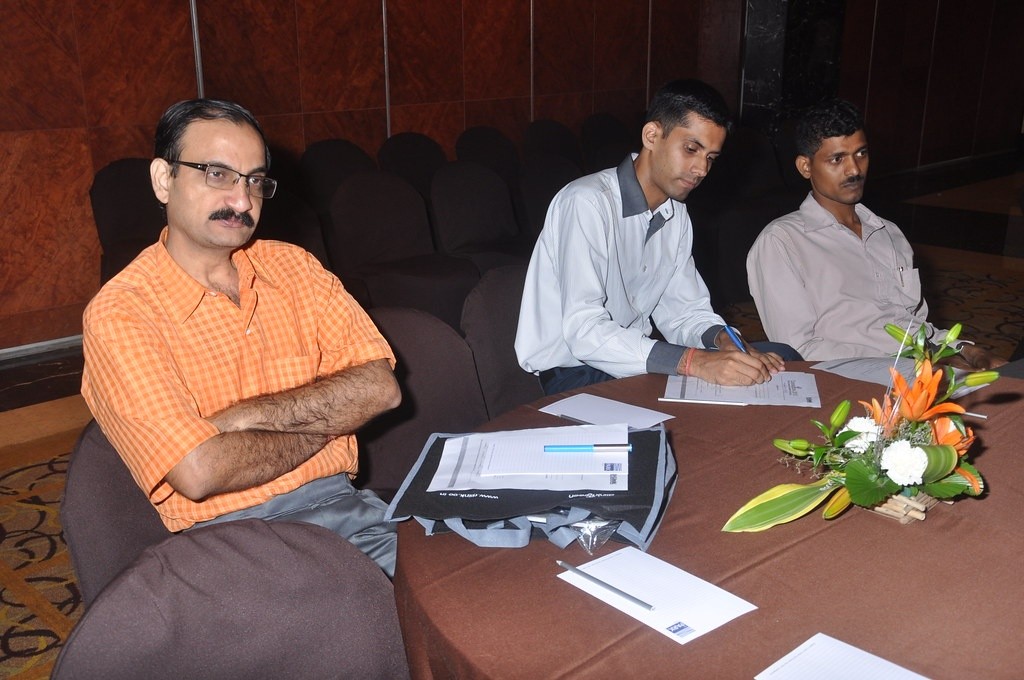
[80,98,400,580]
[514,96,786,405]
[746,95,1012,373]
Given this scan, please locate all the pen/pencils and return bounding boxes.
[544,444,632,453]
[724,325,748,353]
[556,560,654,612]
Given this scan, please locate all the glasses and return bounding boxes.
[165,159,277,199]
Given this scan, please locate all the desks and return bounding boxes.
[393,361,1024,679]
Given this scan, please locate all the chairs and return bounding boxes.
[51,109,810,680]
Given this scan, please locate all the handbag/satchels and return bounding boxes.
[381,420,681,553]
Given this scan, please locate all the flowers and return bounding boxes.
[720,315,999,537]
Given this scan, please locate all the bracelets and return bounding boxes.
[685,347,697,377]
